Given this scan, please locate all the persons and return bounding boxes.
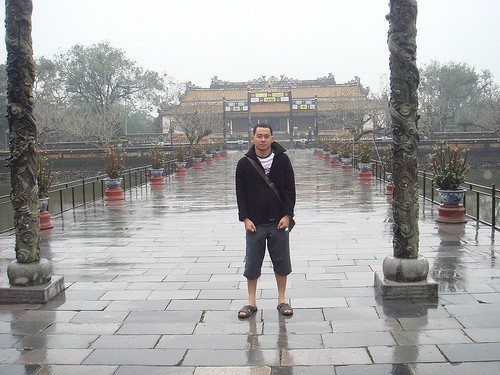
[235,123,296,318]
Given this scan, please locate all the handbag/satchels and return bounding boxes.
[287,217,295,232]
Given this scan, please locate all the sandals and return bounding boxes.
[238,305,256,318]
[277,303,294,316]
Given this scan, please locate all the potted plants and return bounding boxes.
[32,130,474,233]
